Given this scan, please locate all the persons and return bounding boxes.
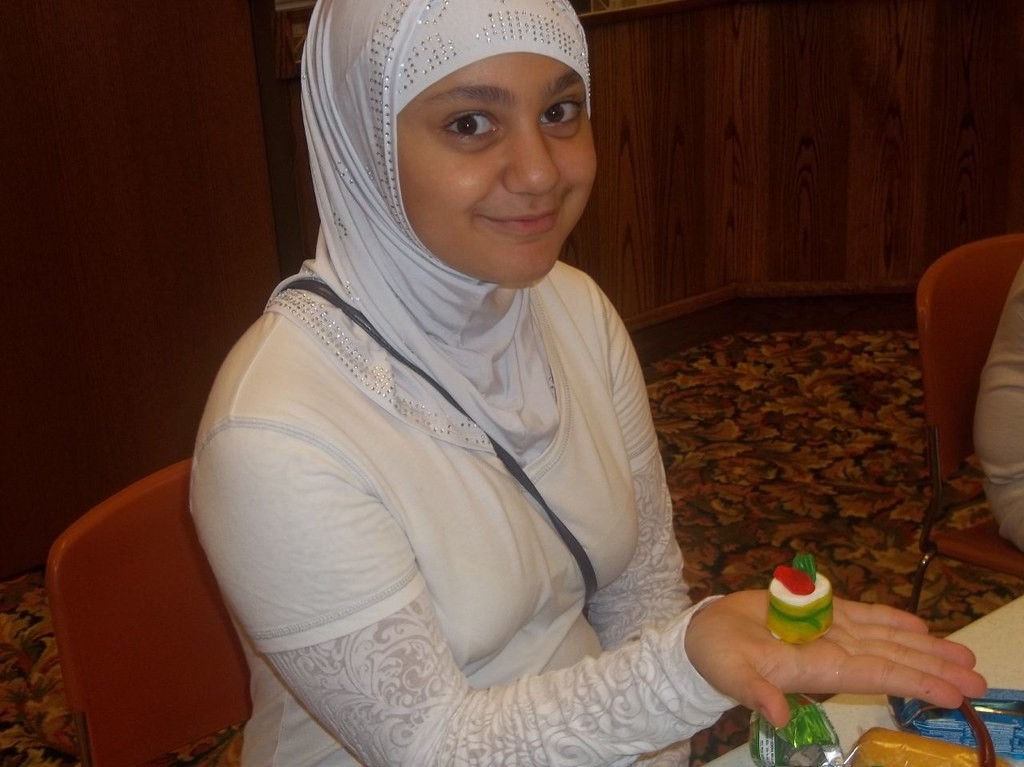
[188,0,989,767]
[975,260,1024,553]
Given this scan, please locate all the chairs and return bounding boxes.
[908,234,1024,613]
[45,458,248,767]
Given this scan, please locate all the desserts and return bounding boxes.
[766,553,833,645]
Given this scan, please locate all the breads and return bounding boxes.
[847,726,1015,767]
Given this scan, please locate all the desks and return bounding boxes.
[701,594,1024,767]
